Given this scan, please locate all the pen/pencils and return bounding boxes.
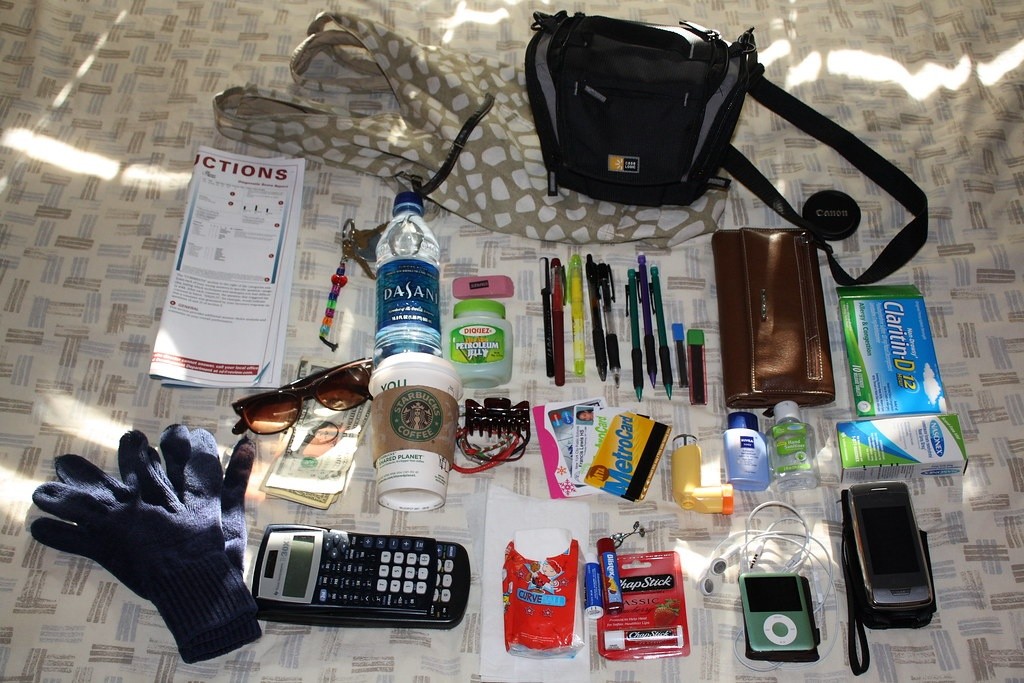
[537,251,675,400]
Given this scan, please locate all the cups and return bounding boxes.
[372,354,462,513]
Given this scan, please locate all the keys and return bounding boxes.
[335,217,392,280]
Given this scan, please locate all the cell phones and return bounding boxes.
[848,481,933,611]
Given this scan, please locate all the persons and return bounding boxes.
[577,410,594,421]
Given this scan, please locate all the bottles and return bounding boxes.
[766,401,817,494]
[374,192,441,356]
[452,300,512,386]
[723,412,770,492]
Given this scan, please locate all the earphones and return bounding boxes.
[710,545,742,575]
[699,569,714,595]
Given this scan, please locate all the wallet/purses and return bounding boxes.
[712,229,836,409]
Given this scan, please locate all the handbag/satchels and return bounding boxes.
[211,11,728,251]
[526,11,929,284]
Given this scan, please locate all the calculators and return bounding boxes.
[250,518,472,632]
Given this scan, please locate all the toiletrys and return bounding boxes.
[724,412,769,491]
[765,401,821,491]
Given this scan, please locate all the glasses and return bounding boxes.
[231,357,374,436]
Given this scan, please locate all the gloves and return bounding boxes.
[30,424,263,664]
